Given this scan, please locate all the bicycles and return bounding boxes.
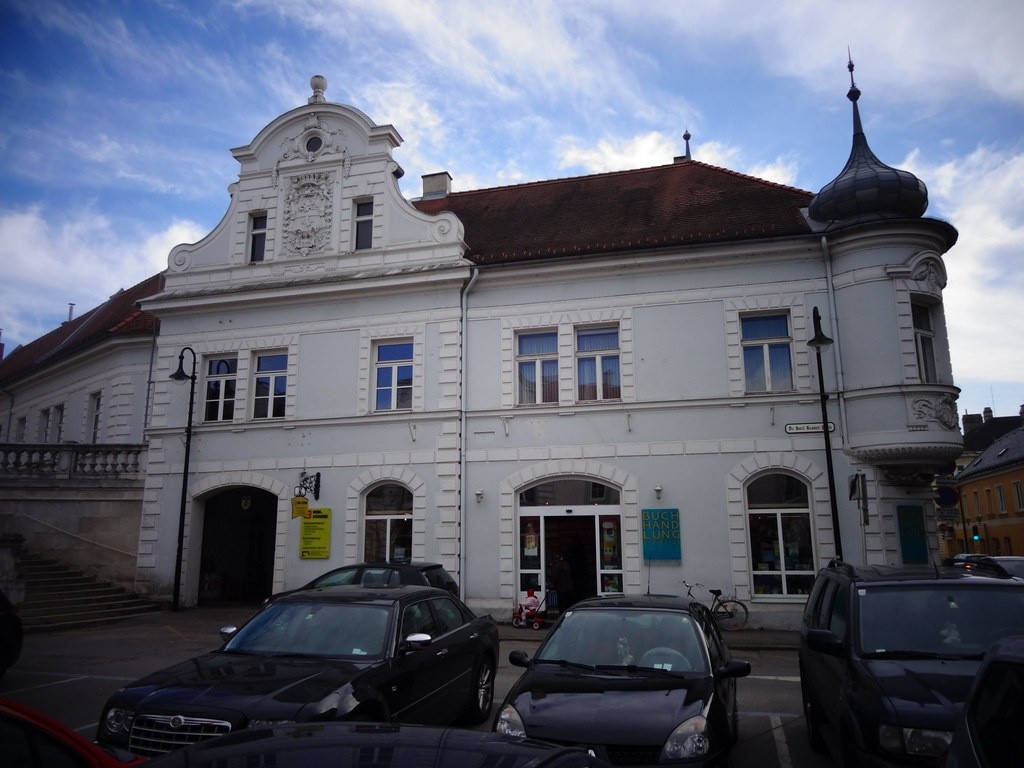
[681,580,749,632]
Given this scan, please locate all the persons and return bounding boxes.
[518,588,539,626]
[550,553,574,615]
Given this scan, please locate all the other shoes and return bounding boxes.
[519,622,526,625]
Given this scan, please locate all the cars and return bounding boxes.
[953,553,990,573]
[263,561,461,606]
[94,582,500,761]
[975,556,1024,579]
[492,594,753,768]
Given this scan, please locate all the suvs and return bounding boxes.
[797,558,1024,768]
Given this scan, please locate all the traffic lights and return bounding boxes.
[972,525,980,542]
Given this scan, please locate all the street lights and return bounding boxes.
[806,305,843,565]
[168,347,196,610]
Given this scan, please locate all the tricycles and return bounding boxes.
[512,604,545,630]
